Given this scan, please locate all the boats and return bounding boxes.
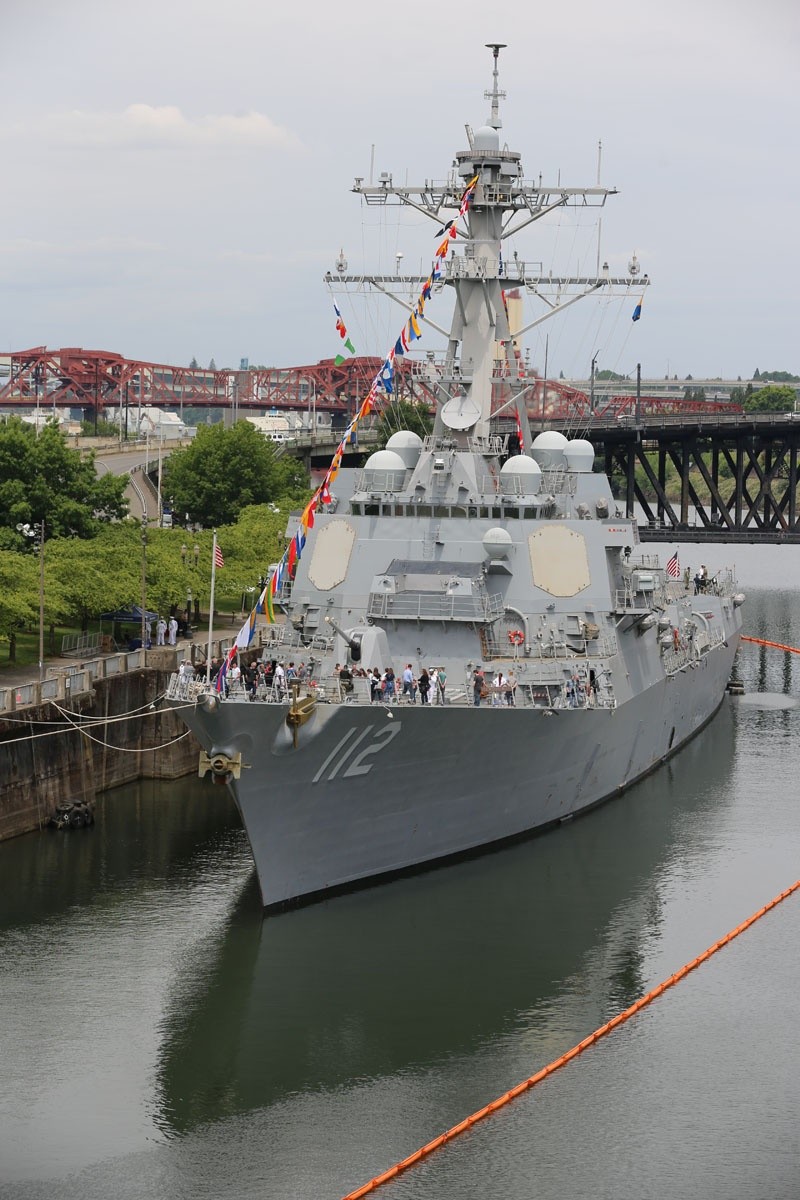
[164,40,747,906]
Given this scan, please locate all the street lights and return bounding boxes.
[140,513,147,647]
[591,347,603,416]
[181,542,200,639]
[300,374,316,437]
[33,519,46,680]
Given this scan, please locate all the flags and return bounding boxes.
[666,552,681,577]
[215,544,225,568]
[333,299,341,318]
[296,377,378,560]
[336,319,347,339]
[631,297,642,321]
[514,407,525,449]
[215,538,297,694]
[334,338,356,366]
[499,290,510,350]
[434,174,479,240]
[376,240,449,396]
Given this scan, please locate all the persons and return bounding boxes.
[466,664,518,707]
[684,564,708,595]
[155,608,189,646]
[564,675,584,708]
[245,660,314,702]
[403,663,448,705]
[178,659,241,699]
[333,663,401,705]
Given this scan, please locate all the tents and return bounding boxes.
[99,603,160,646]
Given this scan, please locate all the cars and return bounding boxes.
[783,411,800,422]
[269,434,296,442]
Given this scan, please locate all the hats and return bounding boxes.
[429,668,435,671]
[170,616,175,619]
